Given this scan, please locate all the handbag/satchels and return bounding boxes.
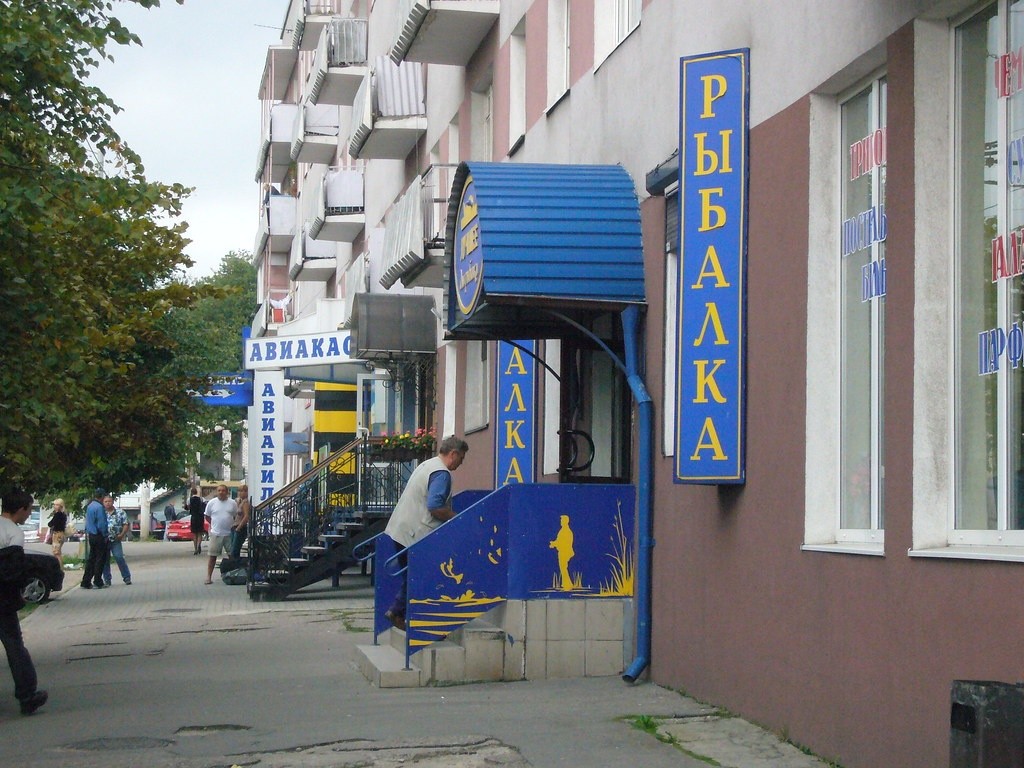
[64,521,76,538]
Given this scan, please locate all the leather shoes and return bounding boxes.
[385,610,407,631]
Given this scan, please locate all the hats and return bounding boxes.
[96,487,106,494]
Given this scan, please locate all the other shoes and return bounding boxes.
[93,579,105,589]
[123,576,131,584]
[21,690,48,714]
[193,547,201,555]
[105,579,111,587]
[204,580,213,585]
[80,581,93,589]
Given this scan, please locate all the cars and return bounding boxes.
[18,549,65,605]
[164,514,212,542]
[130,512,166,540]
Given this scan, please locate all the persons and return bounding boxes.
[204,485,237,584]
[48,498,67,571]
[298,491,310,532]
[80,489,108,589]
[231,485,249,569]
[384,437,471,631]
[187,488,207,555]
[0,491,49,714]
[164,500,177,541]
[103,496,132,587]
[262,184,281,226]
